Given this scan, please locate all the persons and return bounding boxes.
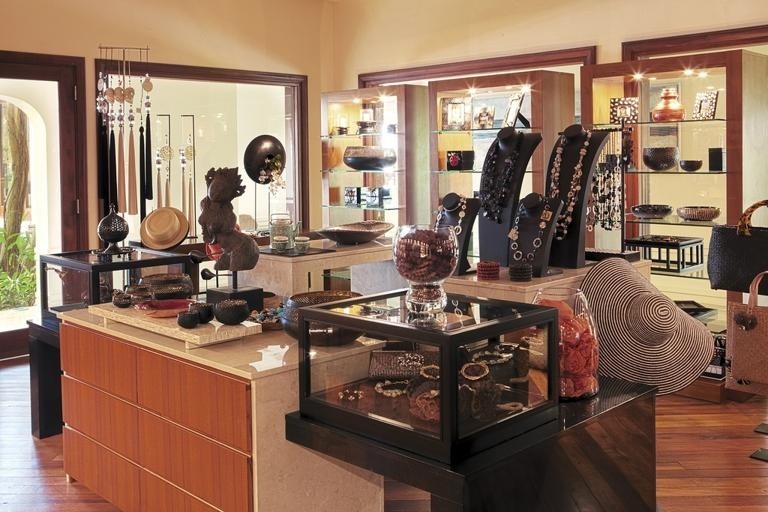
[198,167,259,270]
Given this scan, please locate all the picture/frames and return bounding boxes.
[693,91,718,119]
[501,94,530,128]
[442,97,472,130]
[610,97,638,123]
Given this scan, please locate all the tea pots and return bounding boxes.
[269,213,302,249]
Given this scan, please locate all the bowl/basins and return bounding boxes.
[679,160,704,172]
[178,299,250,328]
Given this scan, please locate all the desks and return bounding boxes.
[228,247,393,298]
[284,375,658,512]
[26,302,85,439]
[56,300,386,512]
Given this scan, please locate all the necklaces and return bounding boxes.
[420,364,440,380]
[508,198,553,264]
[434,194,467,235]
[472,351,513,365]
[375,380,408,398]
[481,131,524,223]
[549,130,592,240]
[461,363,489,380]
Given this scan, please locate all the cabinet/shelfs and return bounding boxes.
[320,132,397,210]
[431,127,532,174]
[591,118,726,279]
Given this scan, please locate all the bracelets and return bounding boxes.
[337,390,364,401]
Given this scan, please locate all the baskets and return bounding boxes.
[313,220,395,245]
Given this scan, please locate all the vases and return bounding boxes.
[652,88,685,122]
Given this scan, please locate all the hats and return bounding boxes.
[140,206,189,250]
[575,257,715,395]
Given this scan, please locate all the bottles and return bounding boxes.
[271,236,309,254]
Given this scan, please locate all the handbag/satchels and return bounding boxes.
[708,200,768,396]
